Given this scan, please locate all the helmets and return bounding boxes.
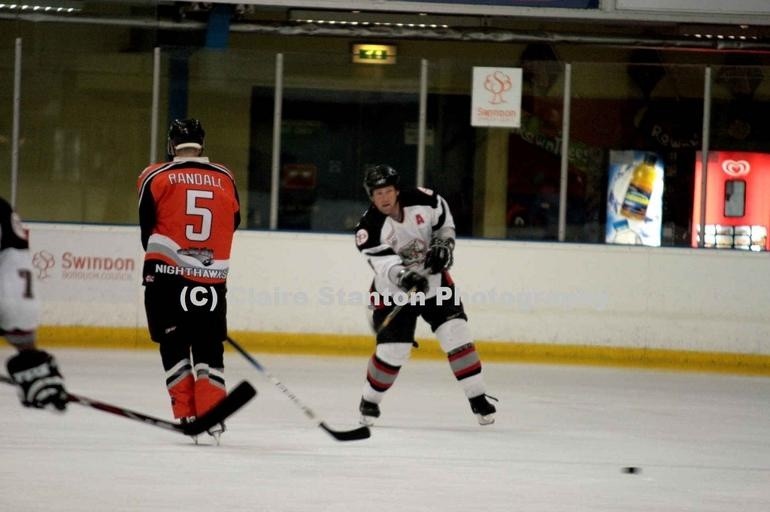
[364,165,399,196]
[167,118,206,150]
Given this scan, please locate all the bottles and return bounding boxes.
[613,152,657,245]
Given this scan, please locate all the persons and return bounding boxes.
[138,115,241,436]
[0,197,68,411]
[355,162,496,417]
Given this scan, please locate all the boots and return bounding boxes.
[180,416,225,435]
[468,393,496,416]
[359,394,381,417]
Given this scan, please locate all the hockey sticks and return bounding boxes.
[1,374,257,436]
[367,266,432,333]
[225,337,371,441]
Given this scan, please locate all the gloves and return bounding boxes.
[395,237,455,298]
[7,349,67,410]
